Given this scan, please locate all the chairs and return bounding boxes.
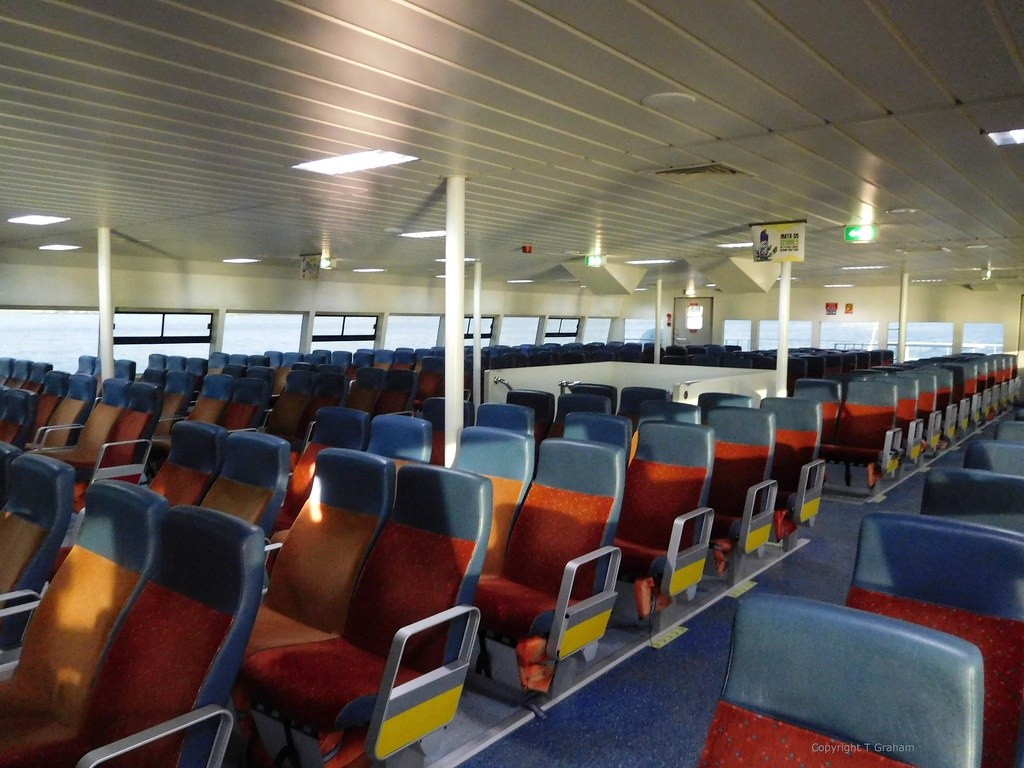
[0,342,1024,768]
